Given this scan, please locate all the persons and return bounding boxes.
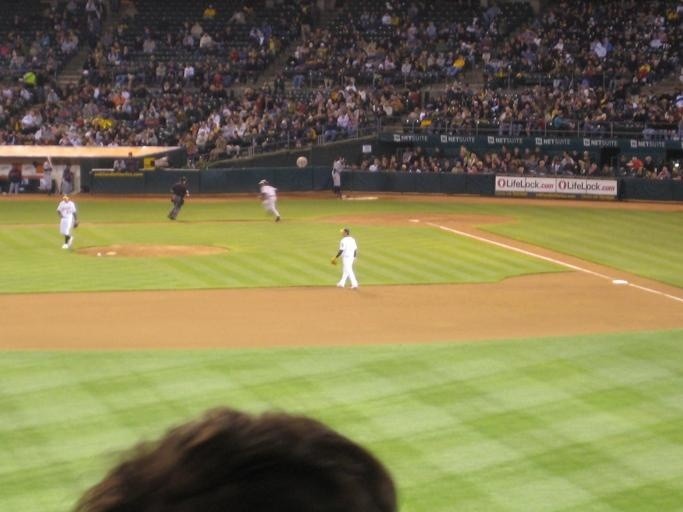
[335,229,358,290]
[0,0,683,194]
[65,406,395,512]
[257,179,281,222]
[57,195,78,248]
[167,176,190,220]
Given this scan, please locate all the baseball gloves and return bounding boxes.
[74,221,79,227]
[331,257,337,264]
